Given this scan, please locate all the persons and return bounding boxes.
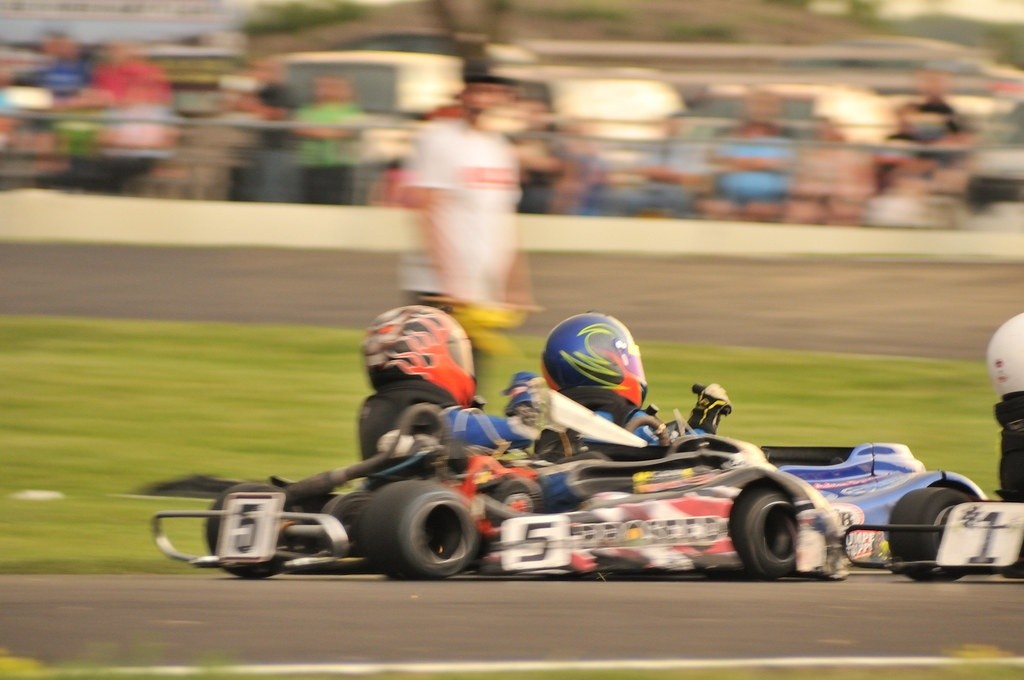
[356,303,732,452]
[400,51,545,371]
[0,28,982,226]
[986,313,1022,500]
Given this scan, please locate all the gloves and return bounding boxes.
[505,372,536,416]
[688,383,731,434]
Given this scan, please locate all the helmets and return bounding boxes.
[542,312,648,408]
[362,304,475,409]
[987,313,1024,397]
